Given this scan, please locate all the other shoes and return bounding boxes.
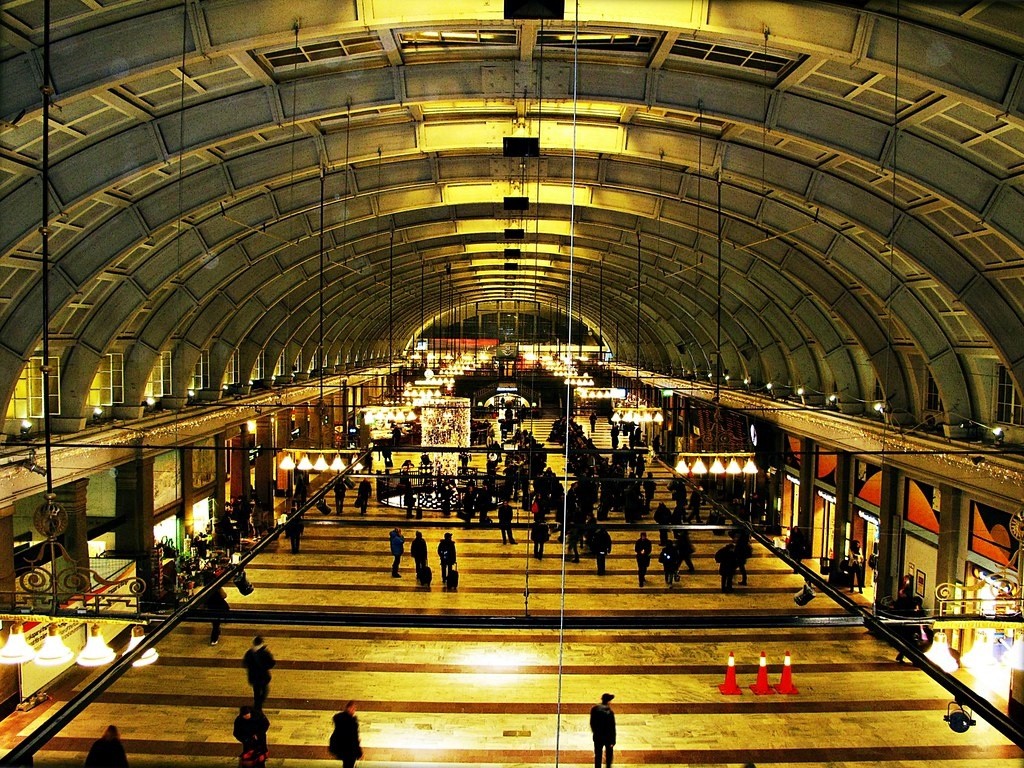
[510,542,518,544]
[738,581,747,586]
[393,573,401,577]
[211,639,218,646]
[572,559,579,563]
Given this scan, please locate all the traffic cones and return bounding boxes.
[718,651,743,695]
[749,650,775,695]
[773,650,800,695]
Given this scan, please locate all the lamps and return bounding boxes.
[575,260,623,399]
[401,257,443,397]
[792,583,818,607]
[361,219,415,423]
[675,171,760,476]
[611,232,664,423]
[0,0,160,668]
[280,169,362,471]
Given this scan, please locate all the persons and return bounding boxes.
[888,590,933,668]
[634,529,697,587]
[435,395,548,545]
[389,528,405,578]
[437,533,457,583]
[202,572,227,645]
[622,471,705,547]
[847,539,865,593]
[524,459,624,576]
[611,423,663,477]
[411,531,427,579]
[232,705,270,768]
[284,508,303,554]
[333,701,364,768]
[714,529,753,594]
[589,693,616,768]
[328,428,419,519]
[545,413,604,478]
[242,636,276,710]
[84,725,130,768]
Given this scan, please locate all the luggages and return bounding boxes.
[447,562,458,589]
[420,559,432,587]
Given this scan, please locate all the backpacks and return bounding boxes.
[743,541,752,558]
[532,503,539,513]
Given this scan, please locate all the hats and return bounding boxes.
[395,527,401,533]
[416,531,421,537]
[602,694,614,701]
[444,533,453,538]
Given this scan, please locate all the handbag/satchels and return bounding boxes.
[240,745,270,768]
[715,550,721,563]
